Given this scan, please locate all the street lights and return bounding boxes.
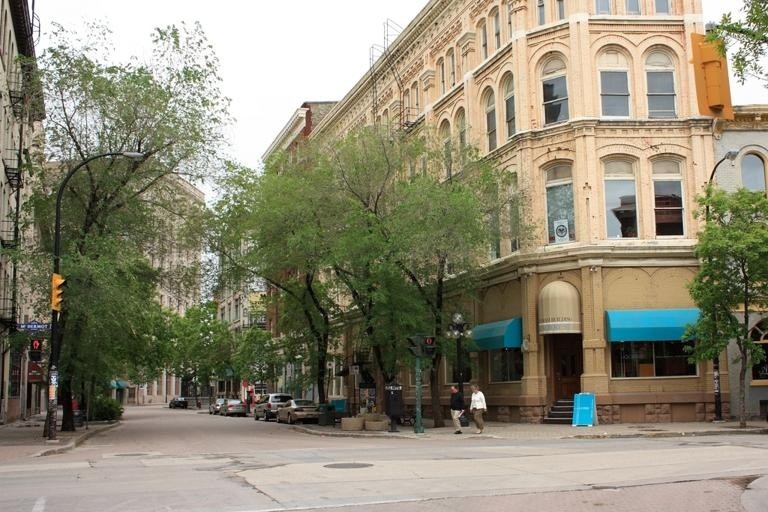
[706,150,741,421]
[446,312,473,427]
[48,152,144,440]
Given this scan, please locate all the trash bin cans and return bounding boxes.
[385,390,402,432]
[760,400,768,419]
[319,404,336,426]
[73,410,83,427]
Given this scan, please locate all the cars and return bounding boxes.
[209,398,247,417]
[169,397,188,409]
[255,393,320,424]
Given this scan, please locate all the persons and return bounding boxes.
[449,382,488,434]
[247,391,252,413]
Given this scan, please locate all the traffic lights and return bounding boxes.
[406,335,438,358]
[51,273,65,312]
